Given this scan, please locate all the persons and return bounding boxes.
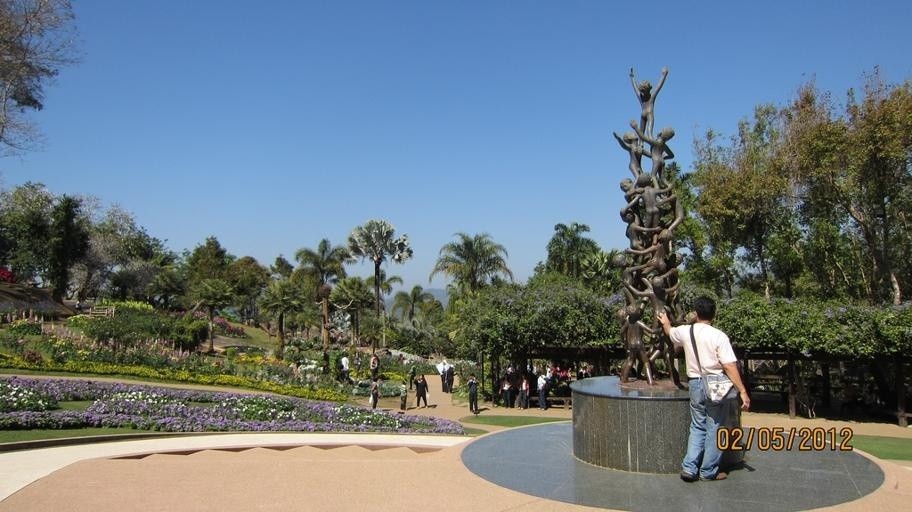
[657,294,752,482]
[435,360,451,392]
[321,345,408,385]
[466,373,480,413]
[371,377,379,410]
[408,360,417,390]
[610,65,684,388]
[499,362,594,411]
[446,365,457,393]
[413,373,429,409]
[399,379,407,414]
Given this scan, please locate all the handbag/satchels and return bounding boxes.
[707,378,739,406]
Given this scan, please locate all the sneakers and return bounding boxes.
[680,469,698,482]
[701,472,727,481]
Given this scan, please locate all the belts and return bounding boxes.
[688,376,702,380]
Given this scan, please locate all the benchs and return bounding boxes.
[893,412,912,427]
[526,395,572,410]
